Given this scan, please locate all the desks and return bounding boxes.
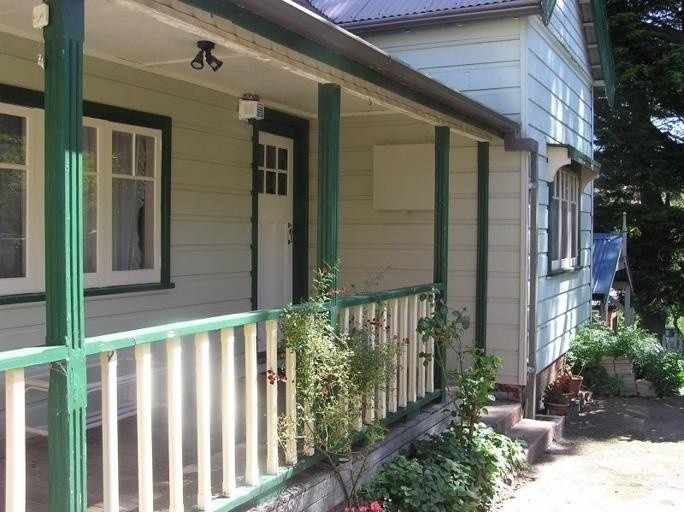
[24,358,167,444]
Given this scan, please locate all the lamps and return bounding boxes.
[188,39,224,72]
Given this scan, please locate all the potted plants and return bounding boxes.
[540,382,569,416]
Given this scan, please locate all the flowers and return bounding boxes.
[554,373,571,391]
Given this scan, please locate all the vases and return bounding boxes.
[562,391,574,401]
[570,374,583,396]
